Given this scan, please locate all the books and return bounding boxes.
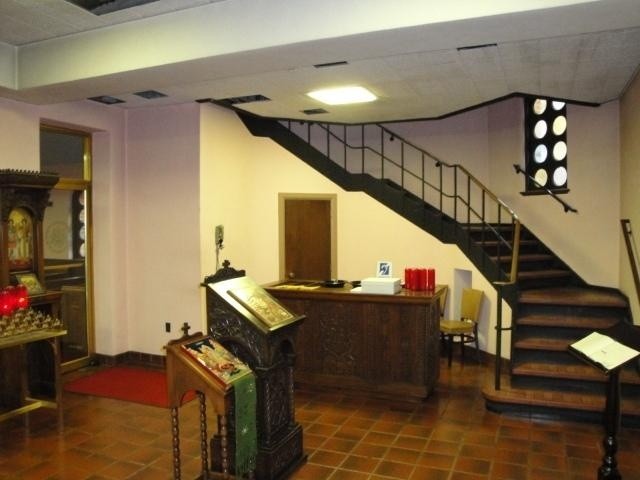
[570,330,640,371]
[179,335,254,391]
[227,283,296,329]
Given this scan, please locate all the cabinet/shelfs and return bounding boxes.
[266,278,447,406]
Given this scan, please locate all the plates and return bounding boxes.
[317,280,347,287]
[350,281,362,287]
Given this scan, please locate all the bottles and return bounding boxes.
[404,267,436,292]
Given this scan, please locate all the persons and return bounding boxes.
[8,218,20,261]
[17,219,32,260]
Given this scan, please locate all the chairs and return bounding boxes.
[439,288,485,368]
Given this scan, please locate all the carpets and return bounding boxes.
[63,364,197,409]
